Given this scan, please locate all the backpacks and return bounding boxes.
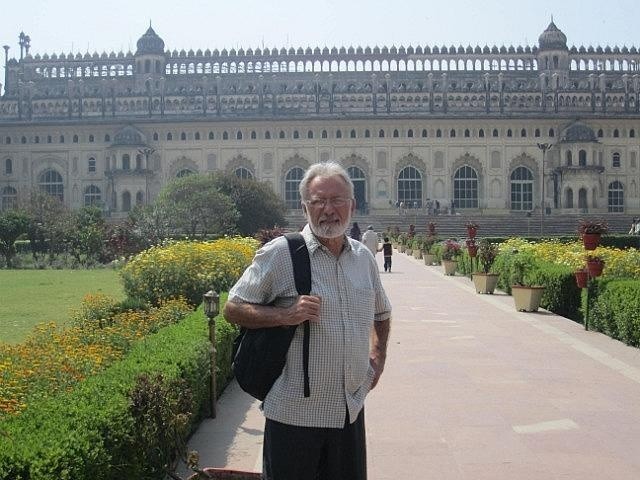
[230,232,311,401]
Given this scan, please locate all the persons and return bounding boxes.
[225,161,391,479]
[393,198,457,217]
[627,216,640,235]
[362,224,379,257]
[350,221,361,240]
[377,237,393,273]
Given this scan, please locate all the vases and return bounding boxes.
[466,225,477,258]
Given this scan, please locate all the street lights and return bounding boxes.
[536,142,553,236]
[137,146,156,208]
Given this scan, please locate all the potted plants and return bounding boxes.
[128,374,263,480]
[472,237,500,294]
[388,227,462,277]
[510,256,546,313]
[574,219,613,288]
